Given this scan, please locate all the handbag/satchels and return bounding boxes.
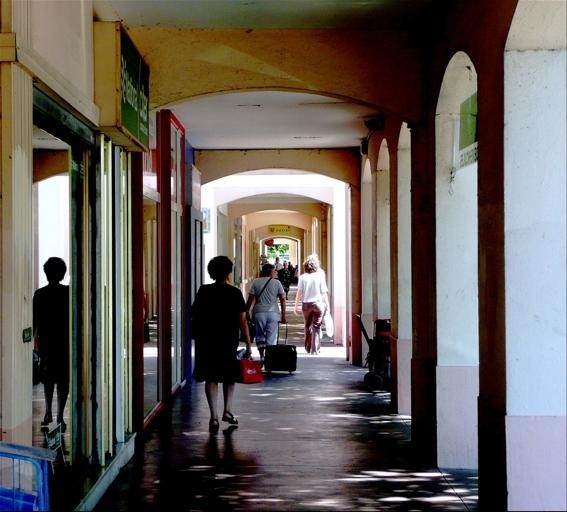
[324,311,334,337]
[239,320,256,343]
[238,355,263,383]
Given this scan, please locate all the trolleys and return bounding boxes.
[356,312,391,388]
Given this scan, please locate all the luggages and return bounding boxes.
[264,321,296,371]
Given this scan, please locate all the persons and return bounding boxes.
[33,257,69,432]
[244,263,286,368]
[293,255,328,353]
[190,256,252,433]
[270,254,299,302]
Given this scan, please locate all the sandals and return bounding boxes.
[210,419,219,428]
[223,411,238,424]
